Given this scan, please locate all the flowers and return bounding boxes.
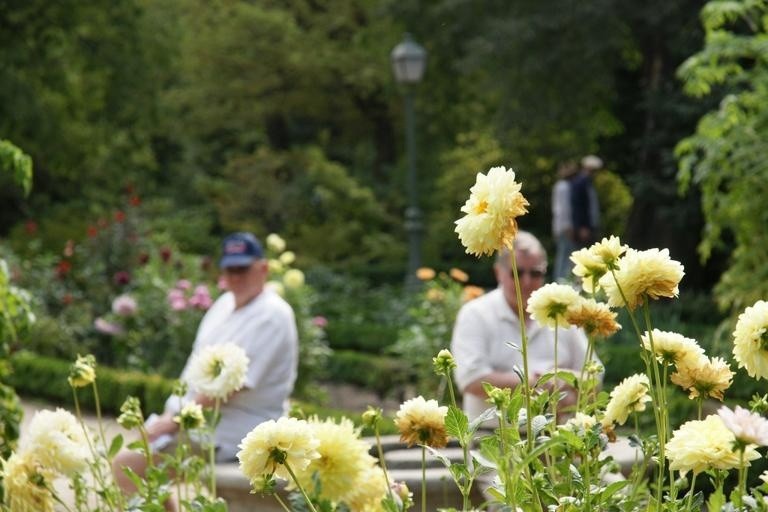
[2,160,768,512]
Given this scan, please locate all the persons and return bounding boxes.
[112,230,301,512]
[452,230,609,512]
[549,158,579,282]
[571,153,605,249]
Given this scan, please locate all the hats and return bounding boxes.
[219,231,263,268]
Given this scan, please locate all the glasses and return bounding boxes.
[504,259,549,280]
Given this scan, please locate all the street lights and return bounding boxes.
[389,31,429,298]
